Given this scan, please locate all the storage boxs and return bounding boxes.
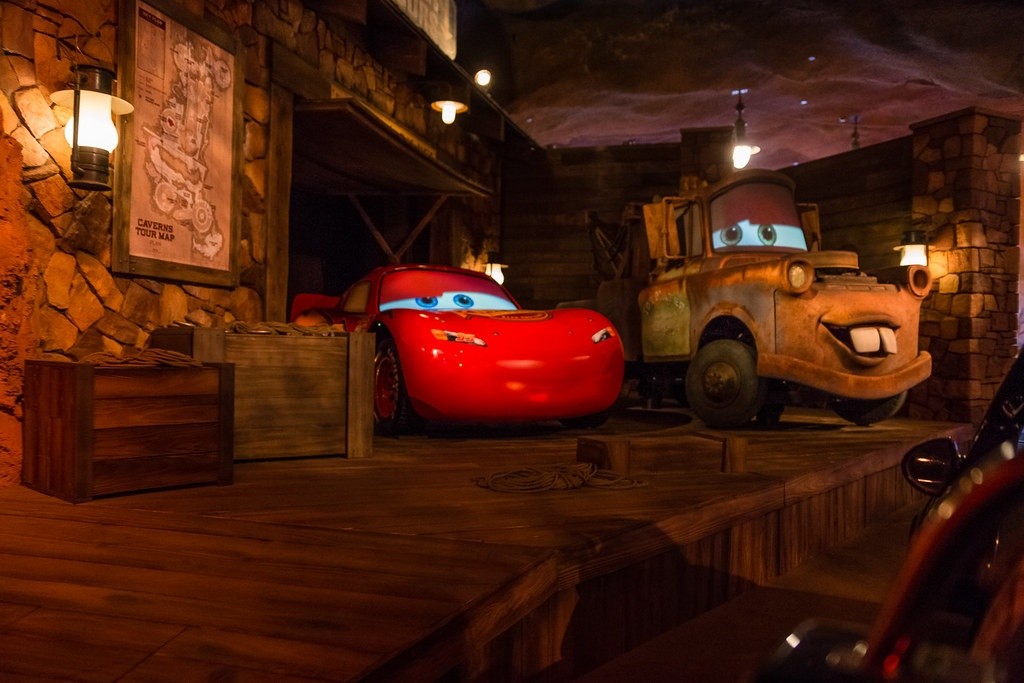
[21,356,236,504]
[152,327,378,471]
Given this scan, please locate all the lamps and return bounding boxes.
[48,29,135,189]
[850,116,861,148]
[893,211,932,267]
[429,85,470,123]
[732,89,761,154]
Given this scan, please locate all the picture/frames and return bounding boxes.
[113,0,244,290]
